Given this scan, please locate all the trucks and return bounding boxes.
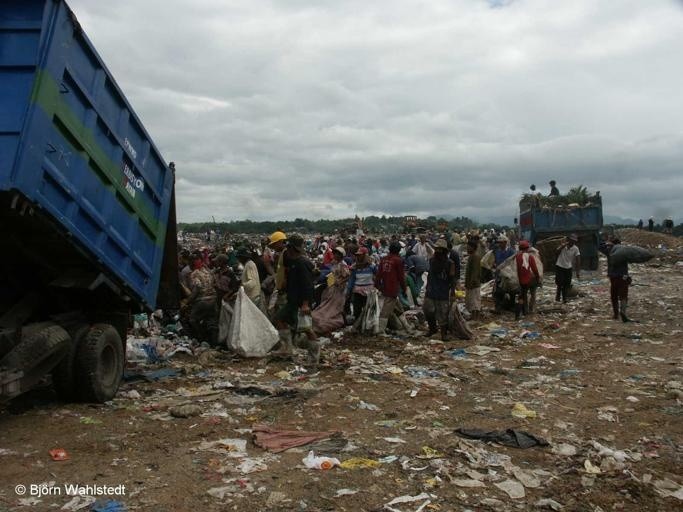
[0,0,181,414]
[511,190,603,273]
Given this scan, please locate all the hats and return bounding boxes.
[331,233,579,257]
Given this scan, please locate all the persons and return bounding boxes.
[173,178,675,362]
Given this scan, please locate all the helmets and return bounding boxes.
[268,232,287,245]
